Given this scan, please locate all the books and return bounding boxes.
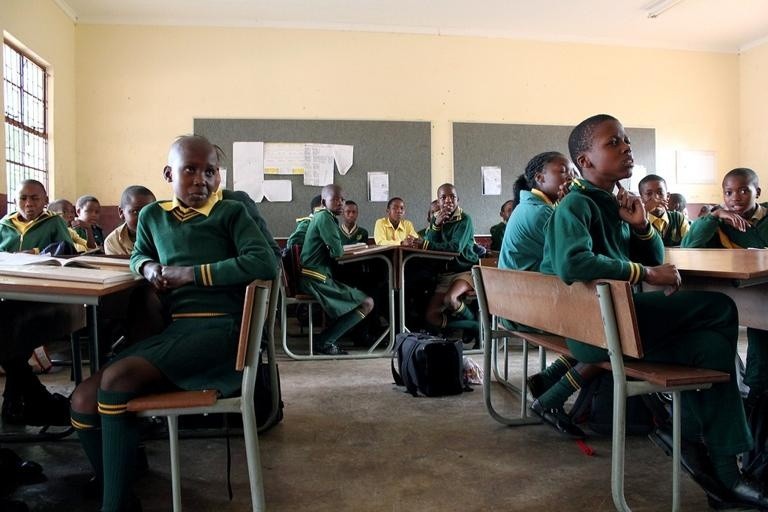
[342,242,368,252]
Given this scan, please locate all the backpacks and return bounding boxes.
[390,332,473,397]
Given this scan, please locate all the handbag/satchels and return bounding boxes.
[463,354,484,385]
[569,367,670,435]
[167,364,284,428]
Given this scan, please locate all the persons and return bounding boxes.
[296,182,376,356]
[373,197,422,310]
[333,198,369,290]
[286,194,326,250]
[424,150,768,448]
[539,113,767,512]
[0,135,288,512]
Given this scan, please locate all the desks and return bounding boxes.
[335,238,458,357]
[657,247,767,328]
[1,250,142,441]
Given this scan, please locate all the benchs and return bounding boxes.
[470,267,729,509]
[278,243,330,363]
[118,270,280,509]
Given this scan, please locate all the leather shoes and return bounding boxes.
[528,398,584,440]
[709,476,768,512]
[462,312,477,344]
[81,465,150,503]
[648,421,731,504]
[313,341,348,355]
[2,393,70,427]
[472,336,480,350]
[1,497,29,512]
[0,448,42,484]
[527,374,547,401]
